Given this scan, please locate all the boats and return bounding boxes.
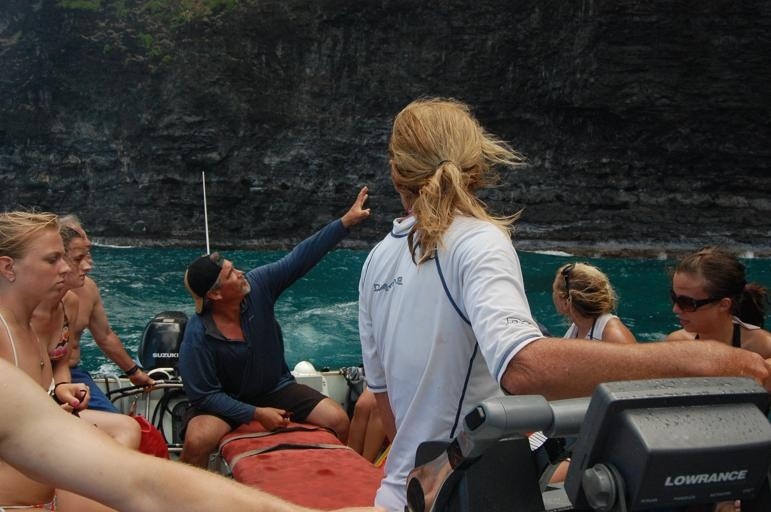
[53,309,769,512]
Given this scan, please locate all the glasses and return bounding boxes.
[560,263,575,300]
[669,289,730,313]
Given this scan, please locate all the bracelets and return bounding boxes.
[53,381,69,394]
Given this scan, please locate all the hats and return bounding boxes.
[183,254,224,316]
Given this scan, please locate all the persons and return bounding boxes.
[0,357,388,512]
[665,248,771,366]
[0,210,119,512]
[548,262,637,483]
[173,185,372,471]
[56,215,156,414]
[348,389,397,463]
[358,99,771,512]
[29,223,142,453]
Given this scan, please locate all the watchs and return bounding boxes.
[125,364,138,376]
